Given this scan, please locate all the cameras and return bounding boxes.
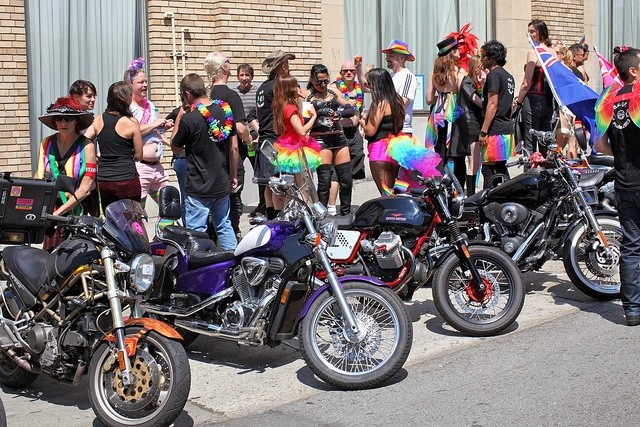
[355,56,360,66]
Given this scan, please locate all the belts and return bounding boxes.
[138,159,162,166]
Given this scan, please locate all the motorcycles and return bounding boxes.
[249,170,525,337]
[488,128,619,219]
[142,140,413,391]
[0,173,191,427]
[393,128,621,301]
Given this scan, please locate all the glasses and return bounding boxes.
[316,80,329,86]
[52,115,78,123]
[342,69,356,73]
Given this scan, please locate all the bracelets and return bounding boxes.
[514,99,526,108]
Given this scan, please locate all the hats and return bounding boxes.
[438,36,460,57]
[38,96,93,130]
[379,40,415,62]
[262,50,295,74]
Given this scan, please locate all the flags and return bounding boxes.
[528,36,602,155]
[596,52,626,90]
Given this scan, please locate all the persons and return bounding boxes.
[301,63,356,217]
[358,67,405,198]
[270,73,323,210]
[327,61,375,217]
[83,81,144,218]
[33,96,97,250]
[256,50,296,222]
[204,51,253,245]
[512,17,558,173]
[353,38,417,138]
[247,106,263,136]
[445,22,480,72]
[566,43,585,161]
[160,83,192,226]
[170,73,239,253]
[577,43,589,85]
[124,57,175,242]
[68,79,100,216]
[231,63,266,218]
[478,39,515,191]
[552,38,576,150]
[426,35,472,193]
[592,45,639,326]
[447,54,490,197]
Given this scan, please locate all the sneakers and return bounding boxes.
[250,204,266,216]
[624,306,640,325]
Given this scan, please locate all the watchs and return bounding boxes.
[479,131,488,137]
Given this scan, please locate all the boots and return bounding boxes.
[316,164,333,208]
[335,162,352,216]
